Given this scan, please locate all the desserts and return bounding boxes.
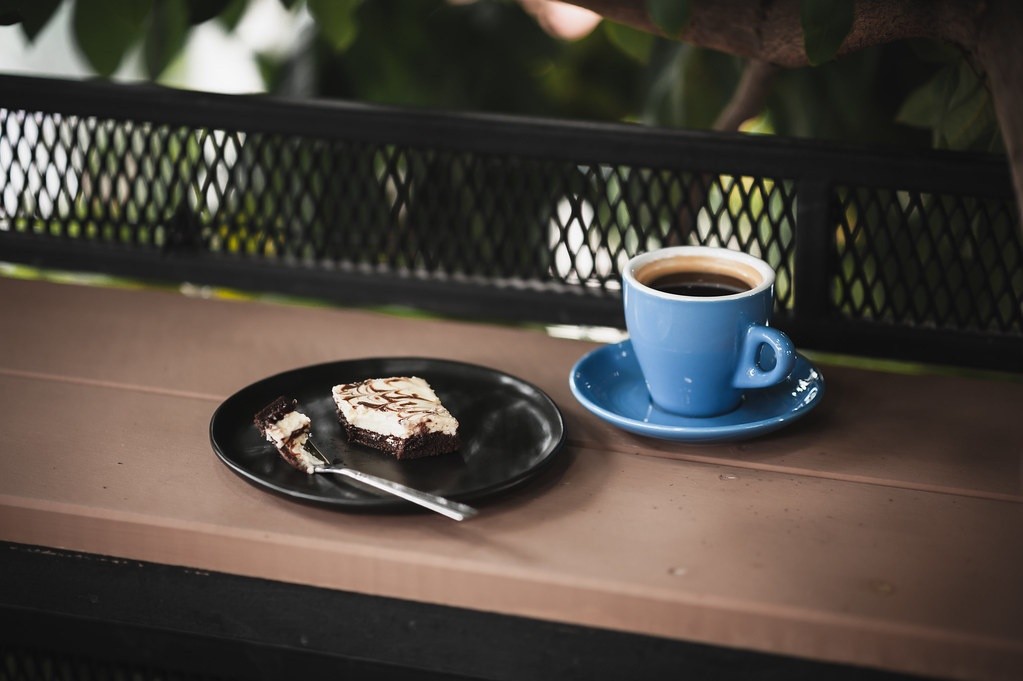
[332,376,461,460]
[251,395,325,472]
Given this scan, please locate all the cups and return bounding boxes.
[623,245,795,416]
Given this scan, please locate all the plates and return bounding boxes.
[572,339,826,444]
[208,357,566,514]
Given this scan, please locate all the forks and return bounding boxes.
[297,439,478,522]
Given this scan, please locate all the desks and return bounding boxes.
[0,272,1023,681]
